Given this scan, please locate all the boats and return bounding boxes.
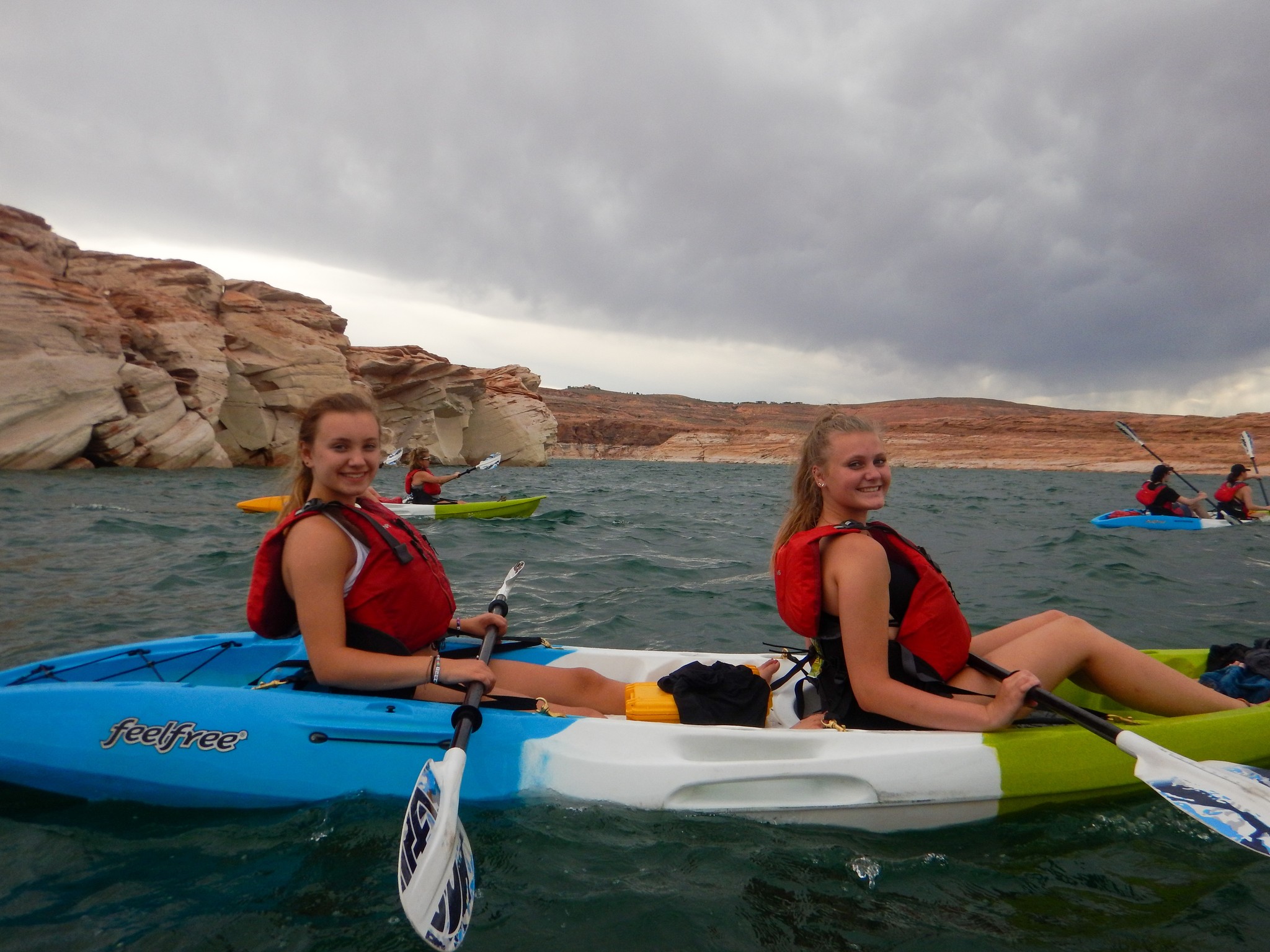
[0,627,1269,834]
[1089,506,1270,530]
[236,493,546,519]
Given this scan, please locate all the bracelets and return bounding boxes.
[426,655,440,684]
[456,618,460,638]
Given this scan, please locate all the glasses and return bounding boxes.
[421,457,431,461]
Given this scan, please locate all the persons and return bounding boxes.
[1214,464,1270,520]
[405,447,467,505]
[247,393,780,728]
[773,411,1257,734]
[1136,465,1213,519]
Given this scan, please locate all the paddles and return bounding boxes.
[396,560,527,952]
[379,447,404,469]
[1239,430,1269,512]
[401,451,501,504]
[1114,420,1242,526]
[965,653,1270,859]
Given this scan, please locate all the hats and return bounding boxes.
[1231,464,1251,474]
[1153,465,1173,476]
